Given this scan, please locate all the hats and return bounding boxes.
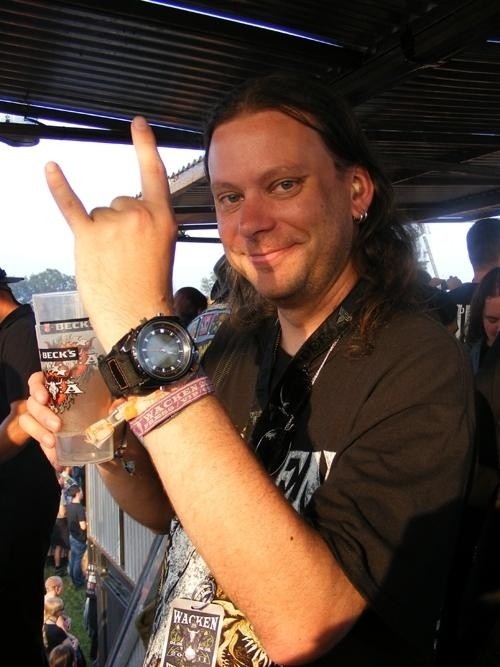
[1,264,26,286]
[209,251,239,300]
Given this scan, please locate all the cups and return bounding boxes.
[30,290,117,466]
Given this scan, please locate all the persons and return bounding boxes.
[2,76,500,666]
